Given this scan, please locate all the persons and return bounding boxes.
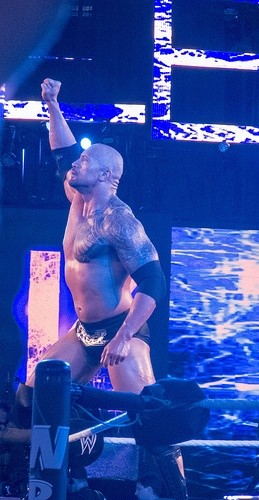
[18,76,188,484]
